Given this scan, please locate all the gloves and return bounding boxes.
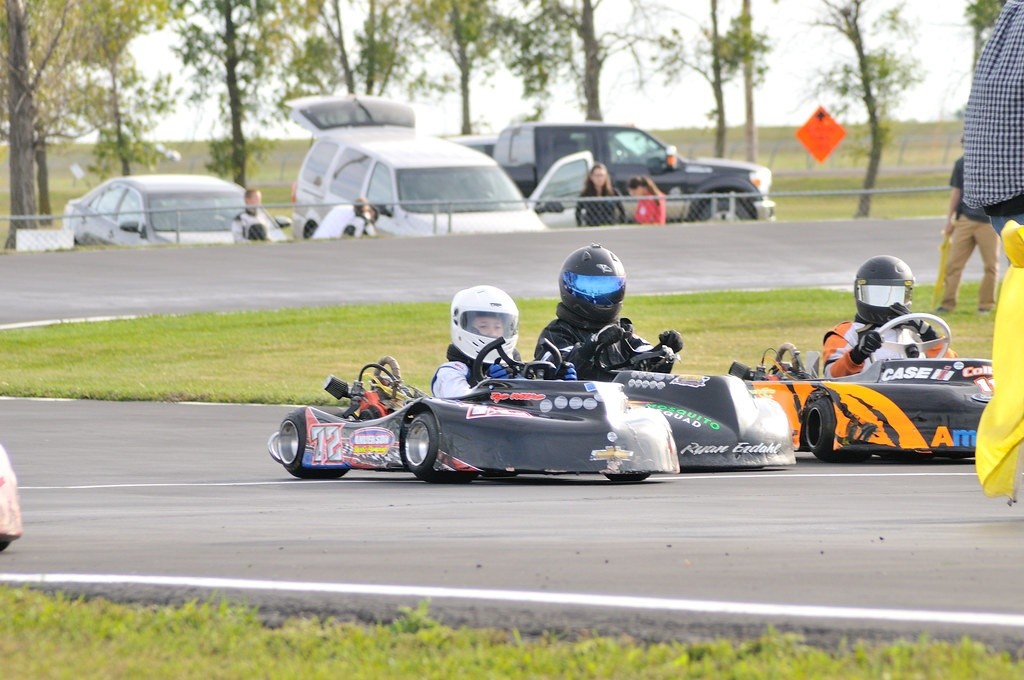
[887,302,934,342]
[586,324,624,346]
[488,364,509,379]
[850,330,882,363]
[562,361,577,380]
[658,329,683,354]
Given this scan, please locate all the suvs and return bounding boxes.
[494,121,777,223]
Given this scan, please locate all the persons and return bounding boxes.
[430,285,577,400]
[819,255,958,378]
[232,188,273,243]
[627,175,667,224]
[575,163,629,227]
[964,0,1024,236]
[534,243,683,383]
[931,137,1001,316]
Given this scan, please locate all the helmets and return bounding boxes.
[450,285,519,364]
[556,241,627,330]
[853,255,916,326]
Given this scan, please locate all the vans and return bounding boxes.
[284,94,596,237]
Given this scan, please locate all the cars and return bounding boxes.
[62,174,292,245]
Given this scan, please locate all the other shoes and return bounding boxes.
[936,306,952,313]
[977,307,993,312]
